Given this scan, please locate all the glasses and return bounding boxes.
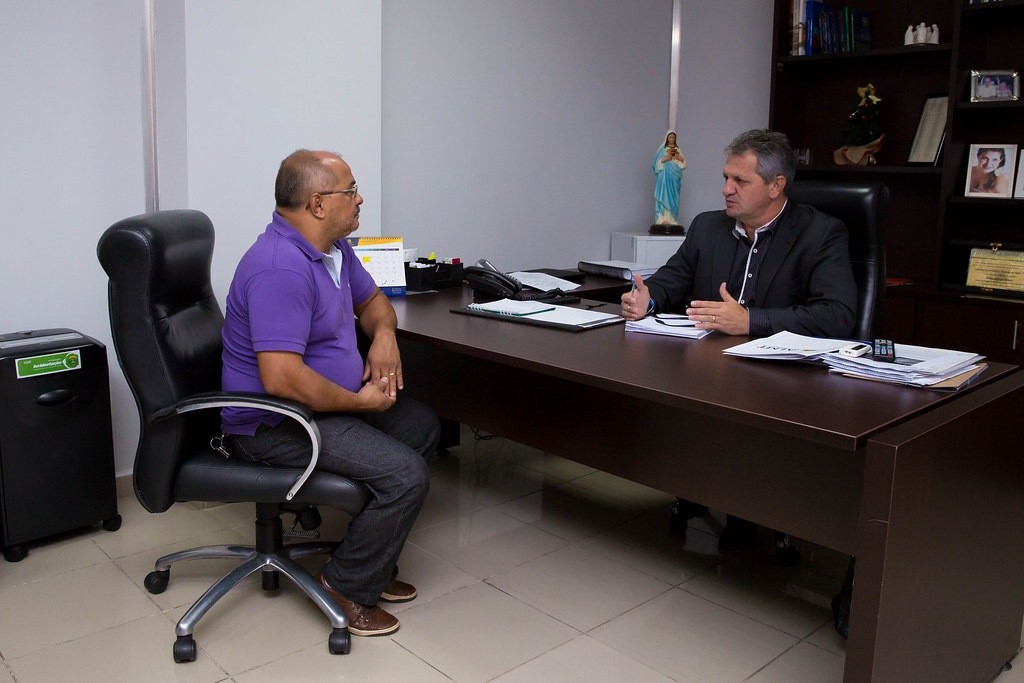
[306,184,357,211]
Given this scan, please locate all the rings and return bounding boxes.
[389,372,395,376]
[627,306,631,311]
[713,315,716,323]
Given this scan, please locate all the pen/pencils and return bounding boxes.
[627,280,635,311]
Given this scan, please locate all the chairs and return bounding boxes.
[772,179,888,569]
[96,209,375,665]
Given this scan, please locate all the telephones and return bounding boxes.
[464,266,523,299]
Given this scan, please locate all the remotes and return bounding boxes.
[873,338,895,362]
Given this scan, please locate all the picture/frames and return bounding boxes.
[903,92,948,167]
[964,144,1018,199]
[970,69,1021,102]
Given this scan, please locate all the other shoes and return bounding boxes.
[670,499,709,527]
[718,514,760,556]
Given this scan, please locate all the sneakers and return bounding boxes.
[379,579,417,601]
[315,568,400,636]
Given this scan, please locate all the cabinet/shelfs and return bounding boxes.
[766,0,1024,366]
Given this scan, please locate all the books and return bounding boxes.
[905,96,949,166]
[788,0,873,55]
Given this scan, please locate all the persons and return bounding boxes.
[222,151,438,637]
[970,148,1008,194]
[653,129,686,225]
[978,77,1011,97]
[904,23,940,44]
[621,130,857,338]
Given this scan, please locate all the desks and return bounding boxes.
[354,284,1024,683]
[522,273,633,304]
